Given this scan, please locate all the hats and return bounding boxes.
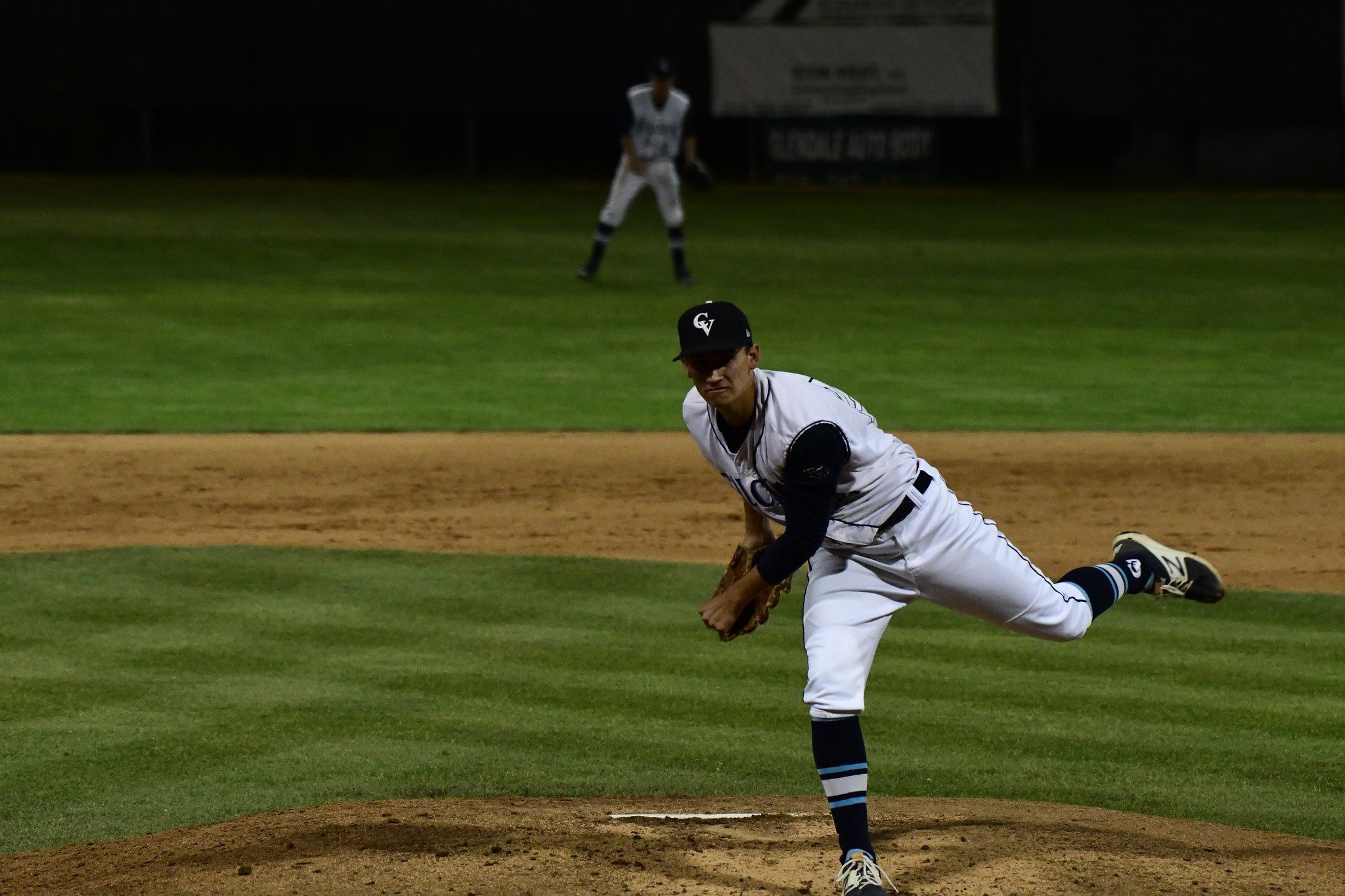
[672,300,752,362]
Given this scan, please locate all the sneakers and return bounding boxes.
[1112,530,1225,604]
[835,849,899,896]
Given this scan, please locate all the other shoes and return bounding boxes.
[675,271,691,282]
[578,264,593,278]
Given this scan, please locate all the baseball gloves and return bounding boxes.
[710,541,795,642]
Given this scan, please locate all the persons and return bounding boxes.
[671,298,1226,896]
[576,62,707,288]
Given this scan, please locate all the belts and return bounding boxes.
[878,471,933,533]
